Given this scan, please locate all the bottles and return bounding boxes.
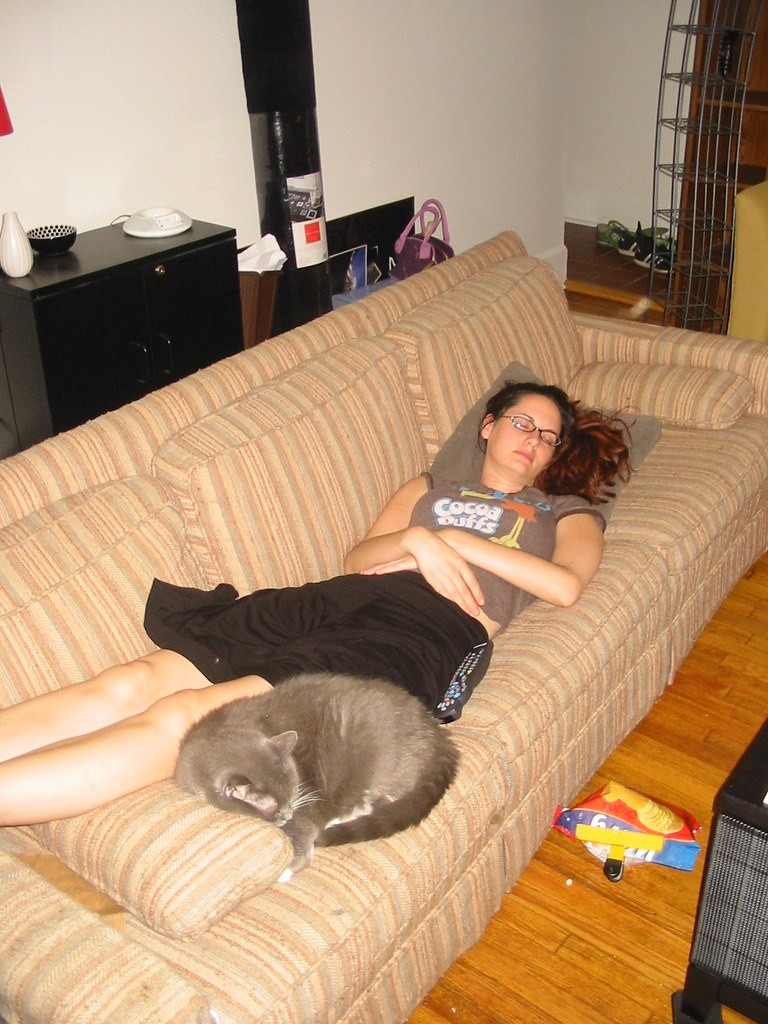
[0,211,33,278]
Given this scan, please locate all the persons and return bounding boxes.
[0,380,638,828]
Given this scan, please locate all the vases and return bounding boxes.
[0,211,34,278]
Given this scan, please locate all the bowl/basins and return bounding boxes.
[26,226,76,255]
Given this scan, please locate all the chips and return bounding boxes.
[639,801,673,832]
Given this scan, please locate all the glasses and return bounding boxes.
[499,416,561,447]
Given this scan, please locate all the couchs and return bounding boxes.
[726,182,768,345]
[0,230,768,1024]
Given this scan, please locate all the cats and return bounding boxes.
[173,673,458,885]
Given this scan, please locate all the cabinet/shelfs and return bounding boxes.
[0,219,247,461]
[645,0,768,331]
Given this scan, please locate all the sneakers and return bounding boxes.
[633,242,675,274]
[617,232,637,257]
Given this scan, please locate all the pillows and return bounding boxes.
[431,361,661,524]
[564,360,756,429]
[28,793,296,937]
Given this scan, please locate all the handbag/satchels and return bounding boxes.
[237,245,284,350]
[388,199,454,282]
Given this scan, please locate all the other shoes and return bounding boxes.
[594,219,677,250]
